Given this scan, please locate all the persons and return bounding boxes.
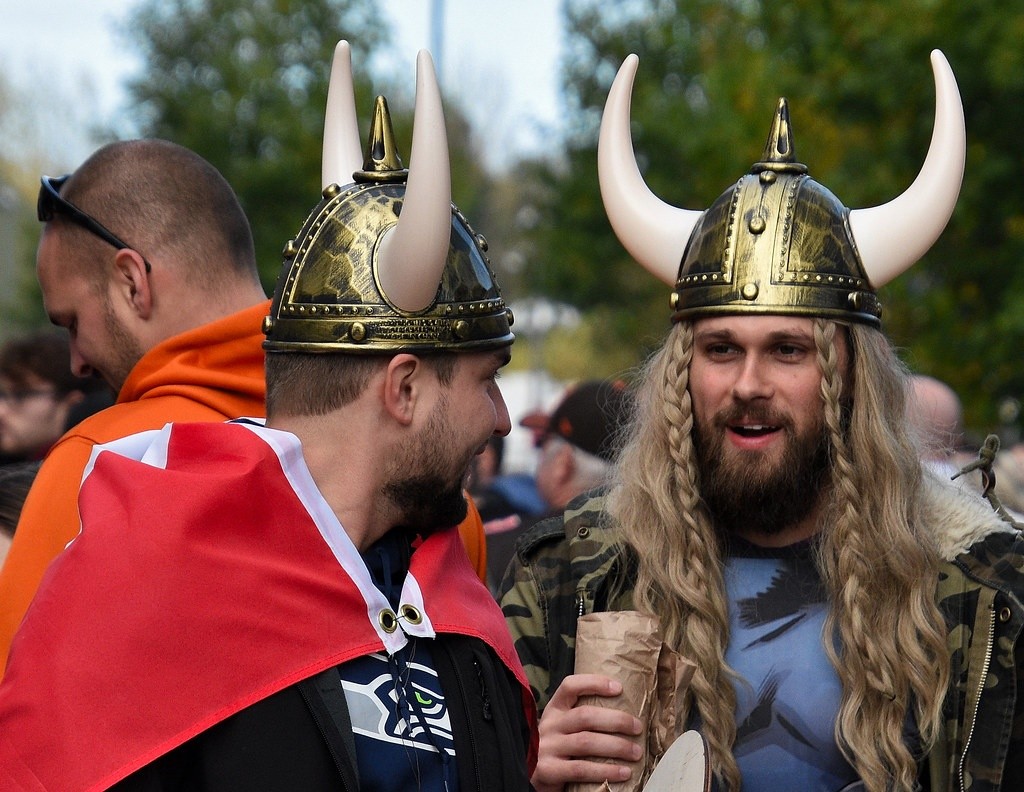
[532,278,1024,792]
[0,331,113,537]
[992,445,1024,511]
[908,375,981,496]
[0,307,543,791]
[472,432,504,483]
[0,137,487,674]
[481,379,636,583]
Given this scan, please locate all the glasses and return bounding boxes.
[35,173,153,275]
[0,383,58,411]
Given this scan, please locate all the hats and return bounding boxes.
[519,378,632,466]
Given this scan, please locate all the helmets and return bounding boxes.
[258,42,517,353]
[596,42,966,326]
